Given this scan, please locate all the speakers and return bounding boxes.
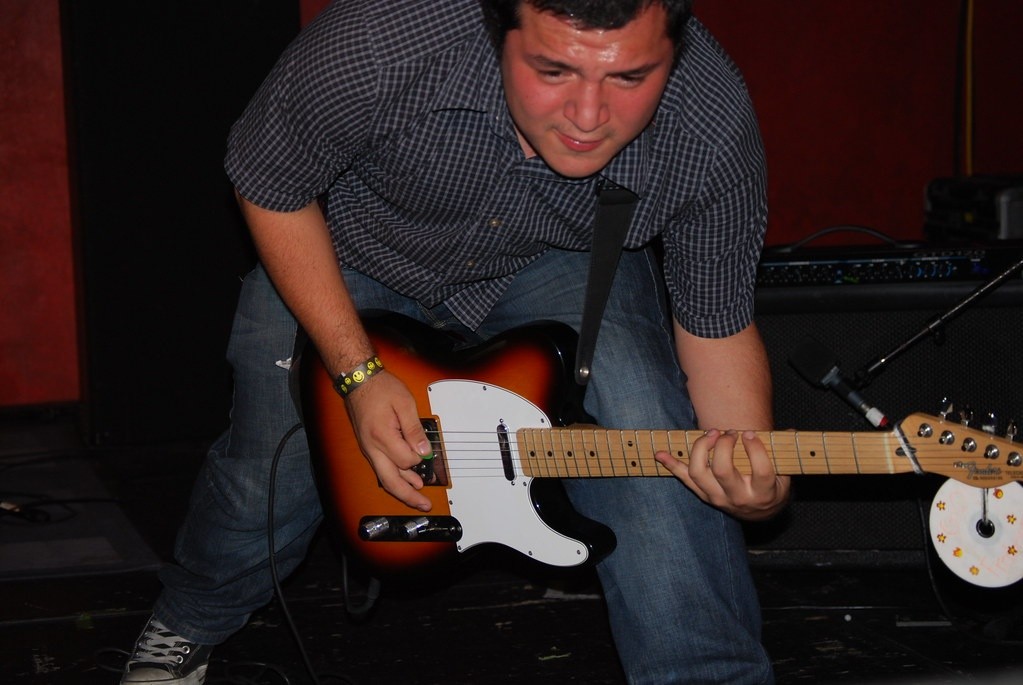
[744,240,1023,568]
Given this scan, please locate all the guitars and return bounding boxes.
[299,310,1023,568]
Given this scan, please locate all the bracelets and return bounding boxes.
[334,355,384,399]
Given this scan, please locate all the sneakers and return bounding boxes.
[120,612,212,685]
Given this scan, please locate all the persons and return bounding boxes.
[120,0,790,684]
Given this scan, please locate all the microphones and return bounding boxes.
[788,335,890,429]
